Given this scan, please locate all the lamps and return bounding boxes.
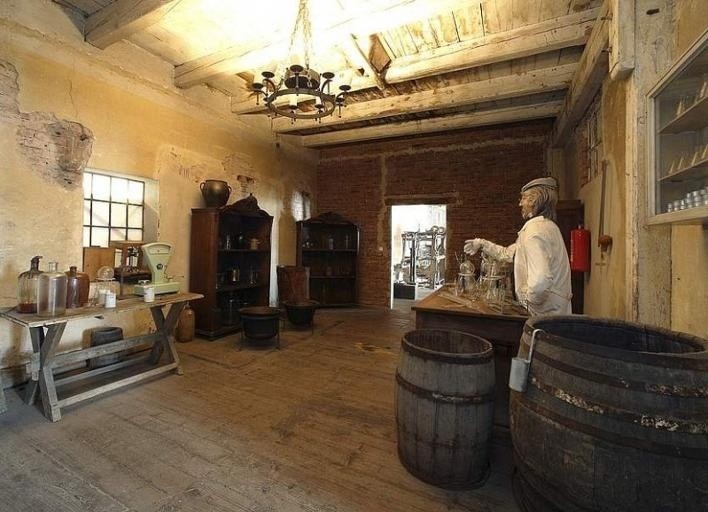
[248,0,353,124]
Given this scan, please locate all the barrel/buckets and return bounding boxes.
[508,315,707,512]
[395,327,497,490]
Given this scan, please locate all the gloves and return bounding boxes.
[464,238,482,256]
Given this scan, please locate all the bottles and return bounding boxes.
[14,255,92,319]
[665,187,708,213]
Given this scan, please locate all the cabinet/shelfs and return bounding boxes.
[295,212,360,308]
[641,26,708,226]
[188,195,274,339]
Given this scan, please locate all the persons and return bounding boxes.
[462,177,574,317]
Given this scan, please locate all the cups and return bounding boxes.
[98,289,108,306]
[104,291,117,309]
[143,285,155,304]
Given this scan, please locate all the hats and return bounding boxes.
[521,177,557,192]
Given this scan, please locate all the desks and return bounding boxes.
[0,292,205,423]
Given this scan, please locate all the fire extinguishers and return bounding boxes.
[570,224,591,273]
